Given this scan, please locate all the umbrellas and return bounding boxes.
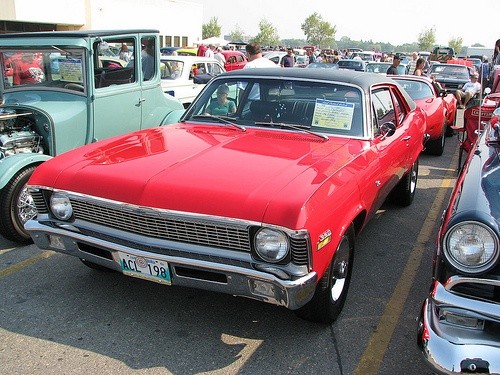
[193,36,231,47]
[229,39,249,45]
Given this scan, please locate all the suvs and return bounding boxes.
[0,28,189,246]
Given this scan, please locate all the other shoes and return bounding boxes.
[457,104,465,109]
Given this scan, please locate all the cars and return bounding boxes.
[49,45,500,158]
[415,107,500,375]
[23,68,429,326]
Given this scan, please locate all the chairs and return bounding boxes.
[450,105,496,173]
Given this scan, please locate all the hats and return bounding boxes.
[394,55,403,60]
[472,72,479,79]
[217,47,222,50]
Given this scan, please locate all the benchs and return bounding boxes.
[245,100,362,130]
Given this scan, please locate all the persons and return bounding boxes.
[237,42,280,110]
[142,37,155,81]
[162,50,179,75]
[119,42,132,62]
[202,84,237,116]
[12,52,46,87]
[452,54,492,109]
[196,44,242,75]
[280,47,294,90]
[307,49,364,71]
[372,51,434,78]
